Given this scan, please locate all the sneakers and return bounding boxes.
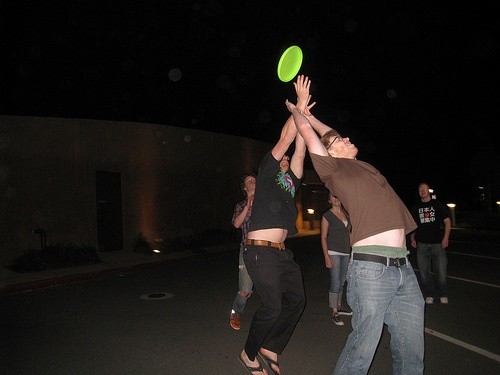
[337,306,352,315]
[230,309,240,330]
[331,313,344,325]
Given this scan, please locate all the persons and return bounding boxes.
[321,193,353,326]
[238,74,316,375]
[230,174,253,330]
[284,98,426,375]
[411,182,451,304]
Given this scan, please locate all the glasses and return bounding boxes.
[326,136,343,151]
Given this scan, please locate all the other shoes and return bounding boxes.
[440,297,448,304]
[426,297,433,304]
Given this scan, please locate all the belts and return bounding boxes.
[246,239,285,251]
[350,252,411,266]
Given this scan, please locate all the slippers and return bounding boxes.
[237,350,281,375]
